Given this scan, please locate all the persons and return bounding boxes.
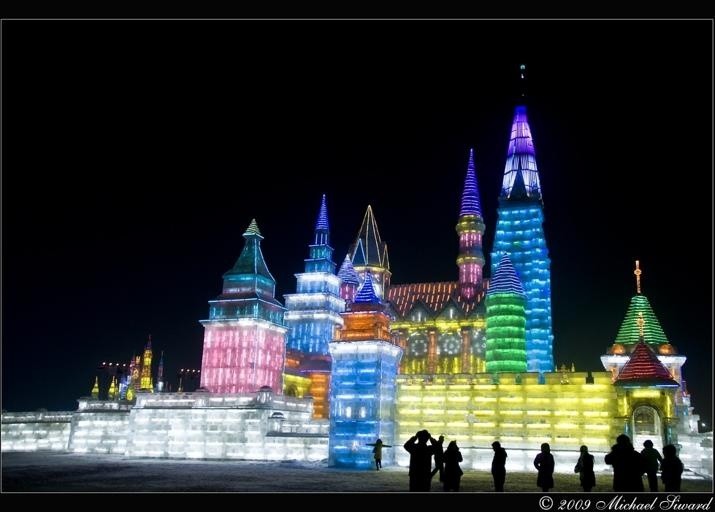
[491,441,509,491]
[661,444,684,489]
[642,439,663,492]
[431,433,445,482]
[574,445,596,491]
[443,440,464,492]
[605,434,645,492]
[404,430,438,492]
[372,439,384,470]
[534,443,557,492]
